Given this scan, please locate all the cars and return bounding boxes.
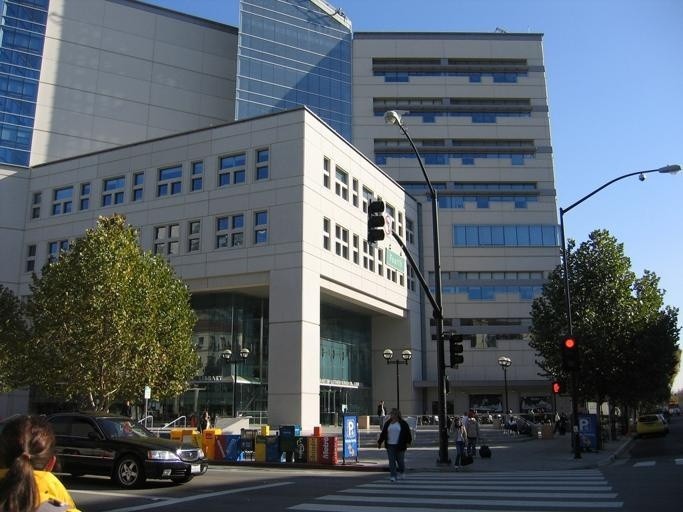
[222,348,250,418]
[36,411,210,490]
[636,414,670,437]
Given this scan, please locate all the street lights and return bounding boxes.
[498,356,512,415]
[383,110,452,464]
[383,348,412,411]
[560,164,682,460]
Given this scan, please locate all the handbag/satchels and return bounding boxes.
[461,455,473,465]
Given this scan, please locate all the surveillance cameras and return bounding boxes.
[639,173,646,181]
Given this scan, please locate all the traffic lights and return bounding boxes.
[367,198,385,243]
[560,334,580,371]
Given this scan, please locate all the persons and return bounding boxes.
[376,401,386,431]
[446,407,567,438]
[199,411,210,436]
[0,414,80,512]
[447,415,466,469]
[376,408,412,482]
[464,411,481,459]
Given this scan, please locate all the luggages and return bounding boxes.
[479,438,492,458]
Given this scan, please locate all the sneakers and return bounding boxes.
[454,465,464,469]
[468,452,476,456]
[390,473,404,482]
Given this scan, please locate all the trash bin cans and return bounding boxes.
[535,425,544,440]
